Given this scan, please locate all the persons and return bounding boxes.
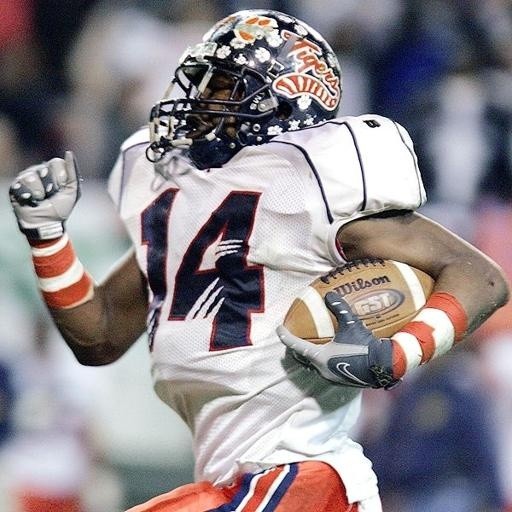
[2,1,510,512]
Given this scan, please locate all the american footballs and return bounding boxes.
[283,256,435,345]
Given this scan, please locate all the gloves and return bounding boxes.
[9,149,84,242]
[276,291,405,390]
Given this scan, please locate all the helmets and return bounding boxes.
[146,8,342,169]
